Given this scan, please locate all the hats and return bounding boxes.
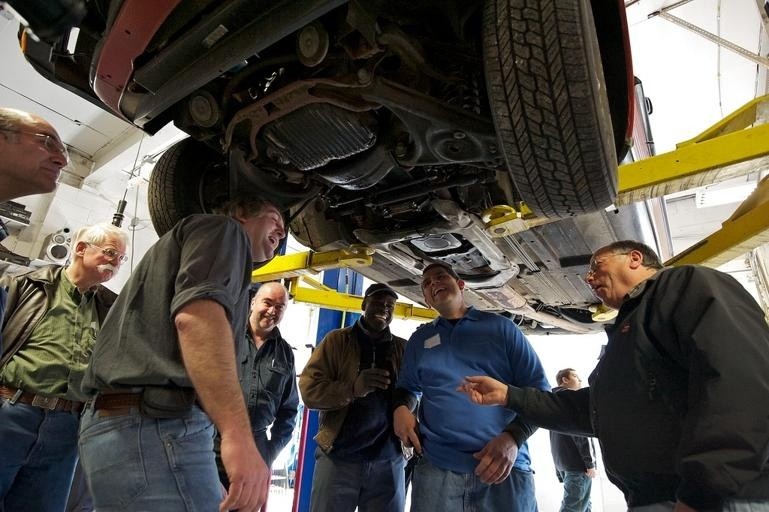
[365,283,399,299]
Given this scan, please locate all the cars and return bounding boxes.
[0,1,676,337]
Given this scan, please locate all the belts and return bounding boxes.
[86,393,141,410]
[0,384,84,412]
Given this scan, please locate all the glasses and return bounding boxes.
[586,252,630,274]
[86,242,128,265]
[0,127,71,164]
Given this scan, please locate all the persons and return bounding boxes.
[298,282,409,512]
[0,106,68,207]
[455,238,768,512]
[0,220,130,512]
[393,262,551,511]
[213,281,299,493]
[548,368,598,512]
[77,193,286,512]
